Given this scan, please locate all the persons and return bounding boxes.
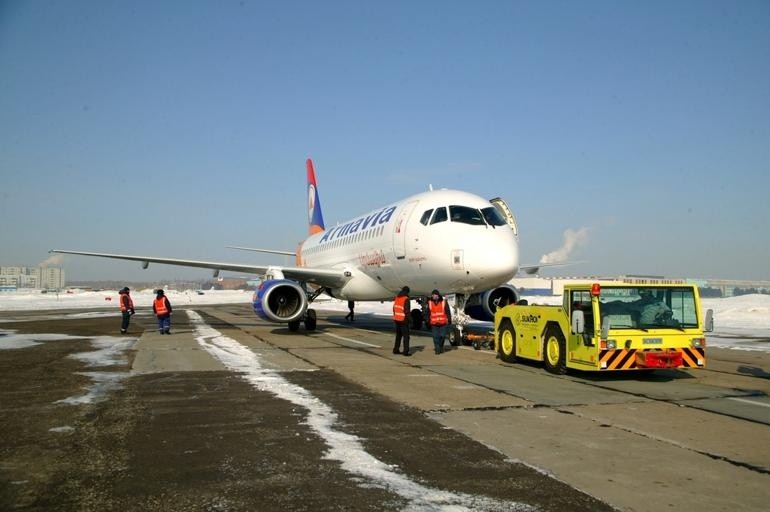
[391,286,413,355]
[344,299,354,321]
[152,289,172,334]
[118,287,136,334]
[424,289,452,354]
[610,289,673,327]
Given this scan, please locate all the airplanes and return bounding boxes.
[49,159,590,347]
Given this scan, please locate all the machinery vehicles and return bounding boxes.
[493,273,715,378]
